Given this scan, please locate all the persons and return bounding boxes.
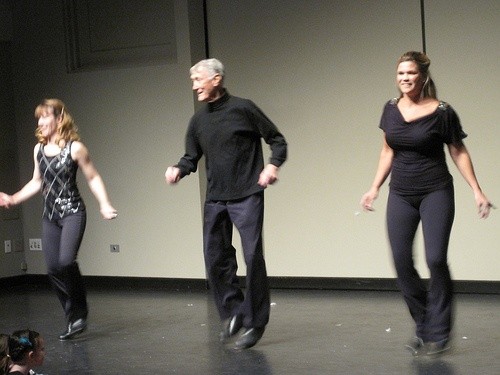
[164,60,289,349]
[0,329,45,375]
[362,53,497,359]
[0,99,117,341]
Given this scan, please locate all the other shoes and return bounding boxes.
[58,317,87,339]
[227,313,243,336]
[403,334,447,360]
[235,324,266,348]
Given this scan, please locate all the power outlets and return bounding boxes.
[29,237,42,251]
[5,240,12,252]
